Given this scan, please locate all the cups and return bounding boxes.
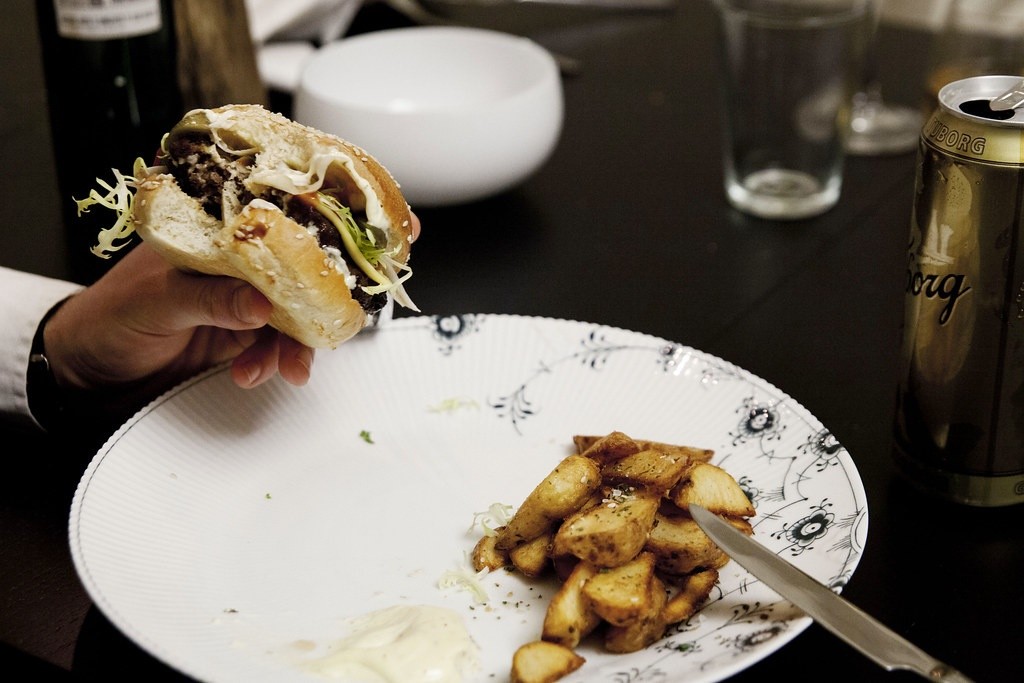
[712,0,1024,218]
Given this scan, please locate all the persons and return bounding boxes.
[0,240,316,432]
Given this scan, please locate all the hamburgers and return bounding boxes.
[72,104,420,350]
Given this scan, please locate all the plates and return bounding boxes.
[69,304,867,683]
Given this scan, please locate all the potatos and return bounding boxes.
[473,431,756,683]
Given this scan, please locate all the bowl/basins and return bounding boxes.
[292,26,564,204]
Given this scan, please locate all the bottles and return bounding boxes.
[32,0,188,278]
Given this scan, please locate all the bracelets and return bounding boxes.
[32,294,80,406]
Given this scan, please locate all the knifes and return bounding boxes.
[687,502,971,683]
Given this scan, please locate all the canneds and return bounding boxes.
[887,74,1024,480]
